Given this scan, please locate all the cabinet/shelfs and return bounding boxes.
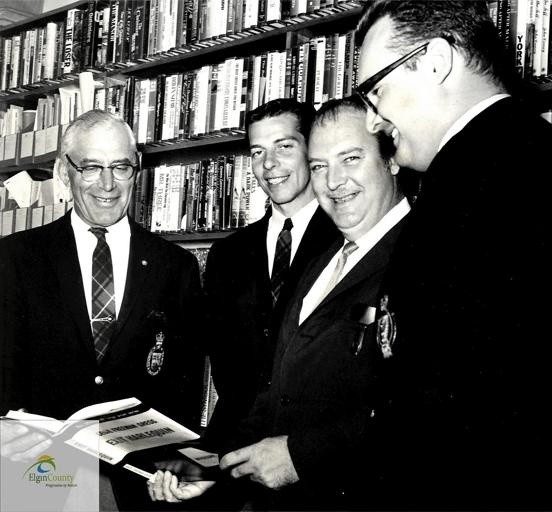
[0,0,551,243]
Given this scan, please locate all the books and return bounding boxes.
[0,1,551,268]
[5,392,228,485]
[198,361,221,428]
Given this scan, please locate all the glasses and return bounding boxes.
[349,36,453,114]
[64,154,140,182]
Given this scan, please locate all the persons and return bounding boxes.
[1,108,204,511]
[202,92,354,511]
[144,92,421,507]
[219,0,550,511]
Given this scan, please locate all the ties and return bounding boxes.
[87,227,117,364]
[321,241,358,305]
[271,219,294,305]
